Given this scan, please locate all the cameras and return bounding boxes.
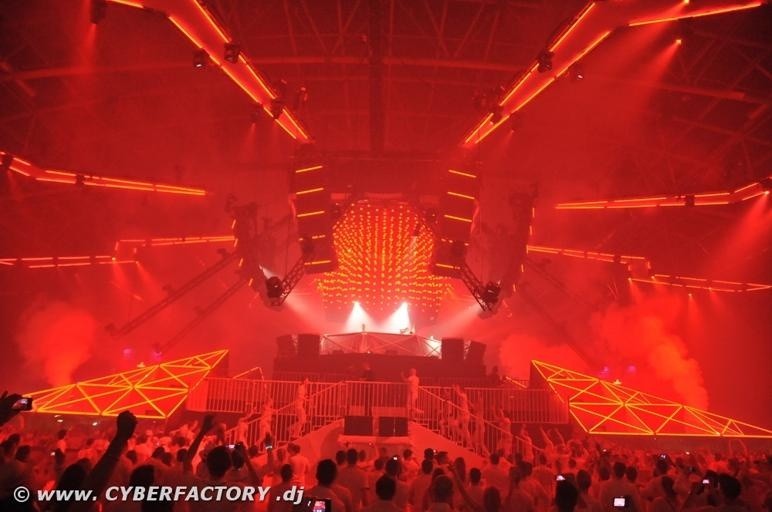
[700,477,712,486]
[660,454,667,460]
[612,495,631,509]
[553,474,566,481]
[226,443,241,450]
[307,498,332,512]
[10,397,33,410]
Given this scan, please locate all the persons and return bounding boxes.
[0,359,772,512]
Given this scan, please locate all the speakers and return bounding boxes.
[343,415,373,436]
[465,340,487,364]
[441,337,464,366]
[379,415,409,436]
[298,333,320,355]
[275,334,296,354]
[529,362,544,388]
